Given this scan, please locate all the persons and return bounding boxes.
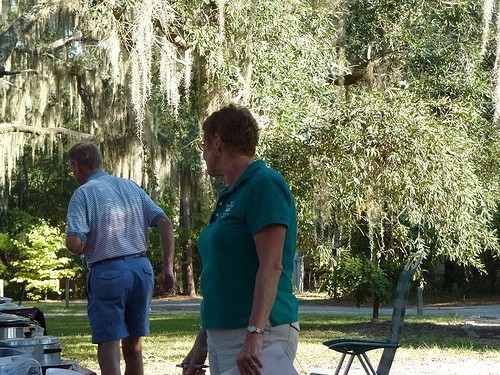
[180,104,300,375]
[66,142,176,375]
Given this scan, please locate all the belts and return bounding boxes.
[94,252,146,264]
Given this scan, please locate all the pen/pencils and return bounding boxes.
[175,362,210,369]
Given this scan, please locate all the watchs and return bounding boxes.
[246,326,263,335]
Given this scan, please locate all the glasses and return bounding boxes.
[197,133,215,151]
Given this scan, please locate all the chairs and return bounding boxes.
[309,254,424,375]
